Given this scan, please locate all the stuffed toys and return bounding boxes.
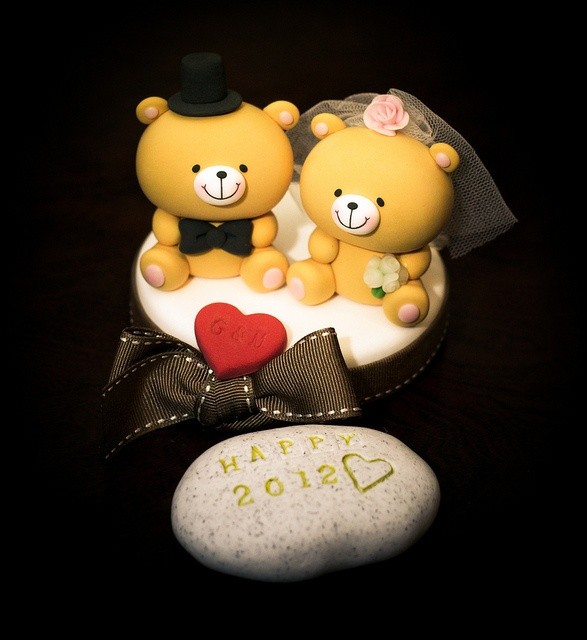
[133,49,300,295]
[285,88,518,328]
